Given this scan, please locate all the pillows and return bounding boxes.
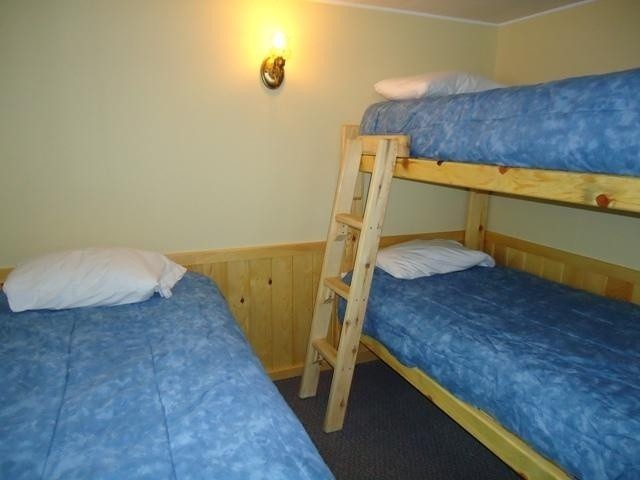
[3,247,187,311]
[375,68,510,101]
[372,237,497,280]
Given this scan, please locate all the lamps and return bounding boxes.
[261,26,286,90]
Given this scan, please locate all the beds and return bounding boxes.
[299,69,640,479]
[0,271,336,478]
